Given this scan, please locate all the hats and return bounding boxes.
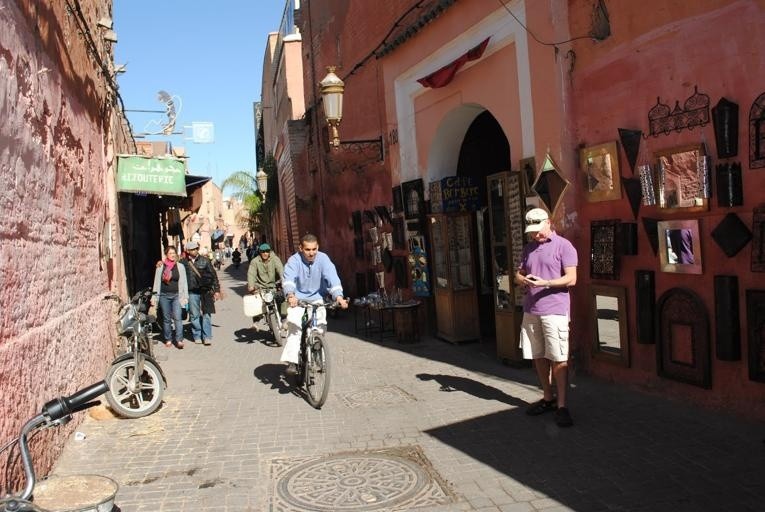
[524,207,550,234]
[185,241,199,250]
[259,243,271,250]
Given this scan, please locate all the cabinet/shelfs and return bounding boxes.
[425,210,488,344]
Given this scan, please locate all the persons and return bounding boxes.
[666,227,694,265]
[205,240,261,273]
[247,243,289,331]
[155,242,220,345]
[150,245,189,349]
[512,207,579,429]
[280,234,352,377]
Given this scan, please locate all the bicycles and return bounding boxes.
[251,276,285,347]
[282,295,351,410]
[0,377,111,512]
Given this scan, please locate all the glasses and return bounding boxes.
[188,247,199,251]
[259,250,270,253]
[523,217,546,225]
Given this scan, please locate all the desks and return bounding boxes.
[348,297,422,346]
[316,61,388,163]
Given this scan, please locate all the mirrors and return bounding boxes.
[576,136,625,202]
[655,143,711,213]
[657,212,708,275]
[587,281,632,370]
[527,148,573,226]
[489,175,517,311]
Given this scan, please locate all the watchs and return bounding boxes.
[546,279,551,289]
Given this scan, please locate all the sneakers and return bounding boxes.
[525,397,558,416]
[284,362,297,376]
[553,404,574,428]
[194,339,202,344]
[280,323,289,338]
[203,338,212,345]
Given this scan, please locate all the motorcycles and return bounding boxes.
[232,254,240,269]
[101,286,170,419]
[213,250,223,272]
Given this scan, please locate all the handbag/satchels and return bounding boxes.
[187,260,214,291]
[146,295,158,323]
[242,289,268,318]
[199,288,216,315]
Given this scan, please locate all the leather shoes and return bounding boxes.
[166,340,172,348]
[175,341,184,349]
